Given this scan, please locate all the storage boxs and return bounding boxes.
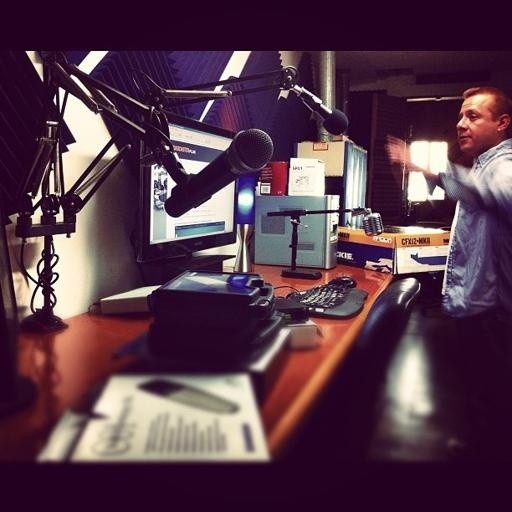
[332,226,452,274]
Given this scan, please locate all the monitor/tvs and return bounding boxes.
[137,108,239,264]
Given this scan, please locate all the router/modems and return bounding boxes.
[100,284,164,315]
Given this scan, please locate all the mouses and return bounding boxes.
[327,277,356,288]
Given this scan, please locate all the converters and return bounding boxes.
[274,297,310,322]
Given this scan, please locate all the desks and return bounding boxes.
[0,245,397,477]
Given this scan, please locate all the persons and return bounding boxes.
[387,85,512,330]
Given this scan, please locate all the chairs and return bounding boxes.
[349,275,424,390]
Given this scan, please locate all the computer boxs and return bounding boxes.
[255,195,340,270]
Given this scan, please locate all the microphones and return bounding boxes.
[362,207,385,236]
[164,127,274,218]
[292,83,349,135]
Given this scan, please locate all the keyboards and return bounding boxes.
[295,283,369,318]
